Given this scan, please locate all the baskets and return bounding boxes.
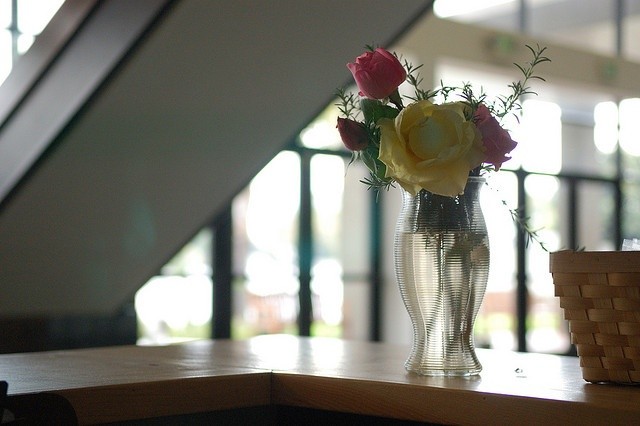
[550,249,640,386]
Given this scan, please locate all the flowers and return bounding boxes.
[330,42,586,364]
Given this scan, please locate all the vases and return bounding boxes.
[393,176,491,378]
